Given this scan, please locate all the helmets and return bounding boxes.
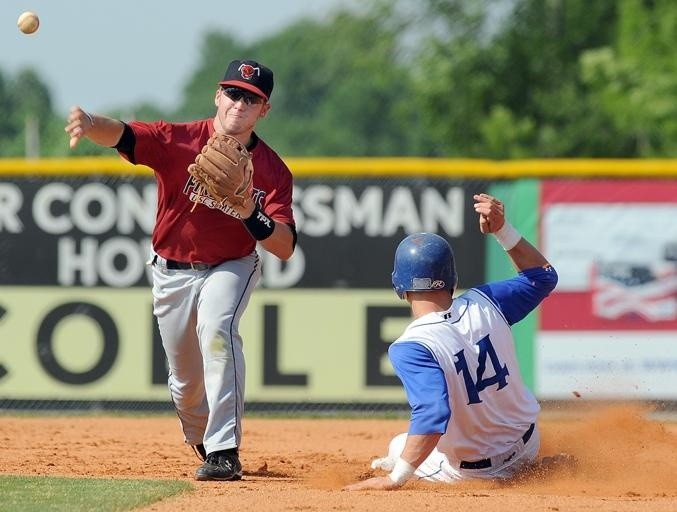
[391,233,457,300]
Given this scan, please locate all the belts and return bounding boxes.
[460,423,534,469]
[166,260,208,273]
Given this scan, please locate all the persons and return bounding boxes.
[338,190,558,490]
[63,58,297,480]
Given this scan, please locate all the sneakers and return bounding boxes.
[192,444,206,462]
[194,450,243,480]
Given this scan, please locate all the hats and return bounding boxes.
[218,59,274,100]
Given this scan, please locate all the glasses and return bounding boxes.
[223,87,262,106]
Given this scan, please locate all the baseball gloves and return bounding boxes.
[187,131,254,213]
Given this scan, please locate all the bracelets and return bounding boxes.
[492,218,522,251]
[85,111,94,129]
[387,457,416,487]
[235,202,275,244]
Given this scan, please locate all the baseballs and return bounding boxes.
[18,11,39,33]
